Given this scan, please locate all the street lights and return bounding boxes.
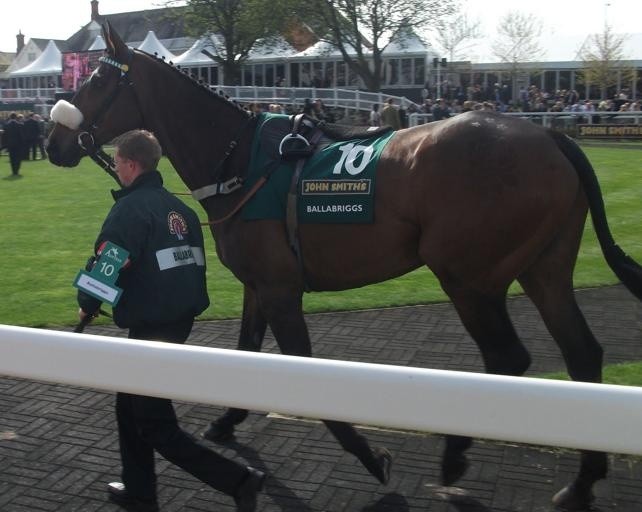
[431,57,447,100]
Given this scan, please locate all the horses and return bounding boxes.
[44,17,641,511]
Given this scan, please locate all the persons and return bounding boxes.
[71,128,266,512]
[5,110,48,175]
[242,81,642,138]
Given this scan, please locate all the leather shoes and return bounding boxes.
[234,466,265,512]
[107,482,129,510]
